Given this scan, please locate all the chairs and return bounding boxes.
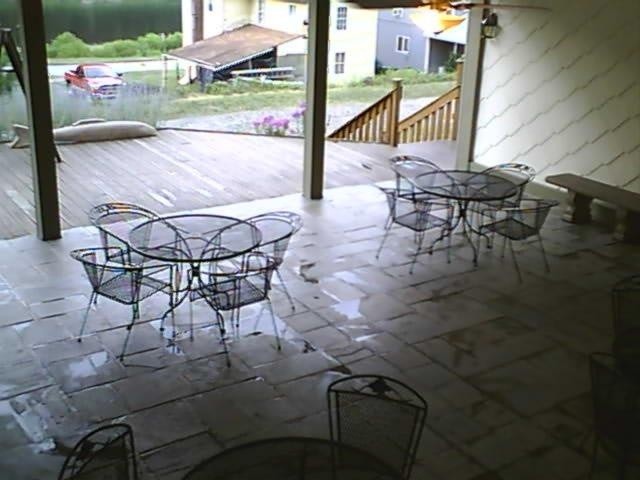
[369,153,561,285]
[55,422,140,479]
[586,271,639,479]
[297,373,429,480]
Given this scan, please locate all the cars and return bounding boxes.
[62,64,128,100]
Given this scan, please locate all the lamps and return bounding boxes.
[478,13,503,39]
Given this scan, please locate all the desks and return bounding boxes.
[178,435,407,480]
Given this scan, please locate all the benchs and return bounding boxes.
[546,170,638,248]
